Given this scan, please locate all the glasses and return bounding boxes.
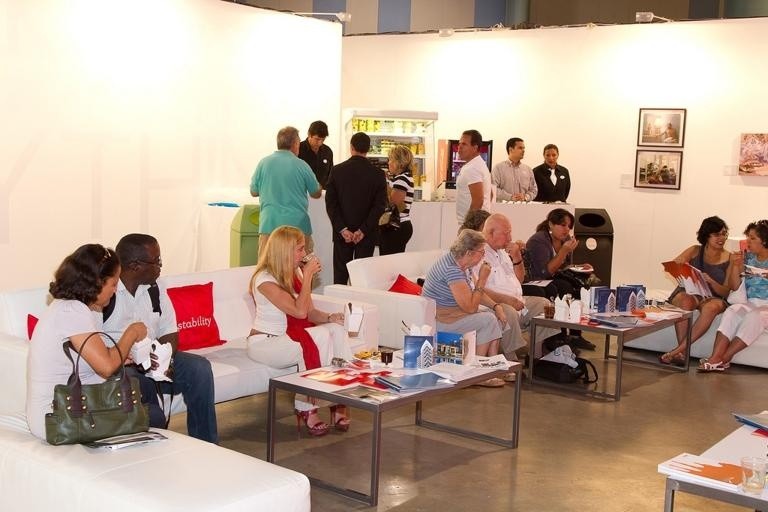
[470,250,486,256]
[711,231,729,239]
[138,255,162,266]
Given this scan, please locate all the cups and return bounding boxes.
[347,311,363,338]
[403,335,433,368]
[543,301,555,319]
[302,253,314,264]
[381,352,392,365]
[740,456,768,494]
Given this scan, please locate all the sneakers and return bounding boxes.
[472,378,506,388]
[573,336,596,350]
[498,370,525,382]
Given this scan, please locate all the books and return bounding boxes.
[658,452,754,492]
[302,358,456,394]
[661,260,713,301]
[645,310,682,319]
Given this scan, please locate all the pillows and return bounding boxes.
[22,312,42,340]
[386,276,426,298]
[162,278,228,353]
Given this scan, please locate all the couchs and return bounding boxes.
[320,249,552,350]
[0,261,381,420]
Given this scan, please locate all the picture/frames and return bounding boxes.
[445,138,492,191]
[632,151,686,190]
[635,104,687,149]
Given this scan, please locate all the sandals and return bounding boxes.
[698,357,731,368]
[696,360,724,372]
[675,351,688,367]
[660,350,674,363]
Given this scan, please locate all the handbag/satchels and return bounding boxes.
[374,203,403,247]
[45,372,150,449]
[533,356,599,387]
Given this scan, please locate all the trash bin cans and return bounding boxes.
[229,204,259,268]
[575,208,613,288]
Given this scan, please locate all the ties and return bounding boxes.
[548,169,558,187]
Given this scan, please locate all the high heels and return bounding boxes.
[293,406,329,436]
[329,404,352,433]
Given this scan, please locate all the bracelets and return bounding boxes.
[328,313,334,322]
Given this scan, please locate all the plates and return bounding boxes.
[352,353,382,362]
[568,266,593,273]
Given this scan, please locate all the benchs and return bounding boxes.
[616,235,767,374]
[0,405,312,512]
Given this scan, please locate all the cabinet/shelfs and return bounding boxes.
[341,109,440,201]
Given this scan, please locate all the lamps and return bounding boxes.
[634,10,674,25]
[291,10,353,25]
[437,27,479,37]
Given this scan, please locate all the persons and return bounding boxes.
[250,126,322,265]
[419,210,561,387]
[297,120,334,189]
[655,216,741,366]
[385,143,415,253]
[456,130,491,236]
[663,122,678,139]
[491,138,538,202]
[26,242,166,441]
[101,234,218,447]
[246,225,350,437]
[325,132,387,285]
[525,208,596,350]
[696,219,768,372]
[533,144,570,203]
